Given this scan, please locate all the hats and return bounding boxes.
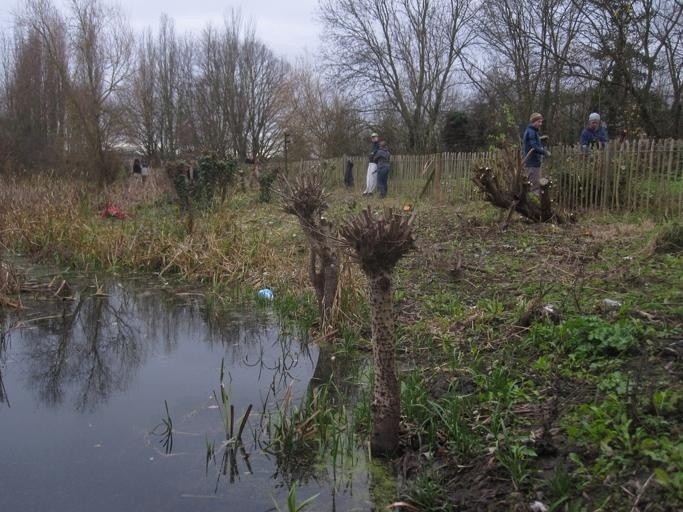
[379,140,386,147]
[370,132,378,137]
[588,112,600,121]
[529,112,543,123]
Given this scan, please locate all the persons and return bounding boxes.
[579,112,609,150]
[371,141,390,197]
[362,131,379,196]
[523,112,551,200]
